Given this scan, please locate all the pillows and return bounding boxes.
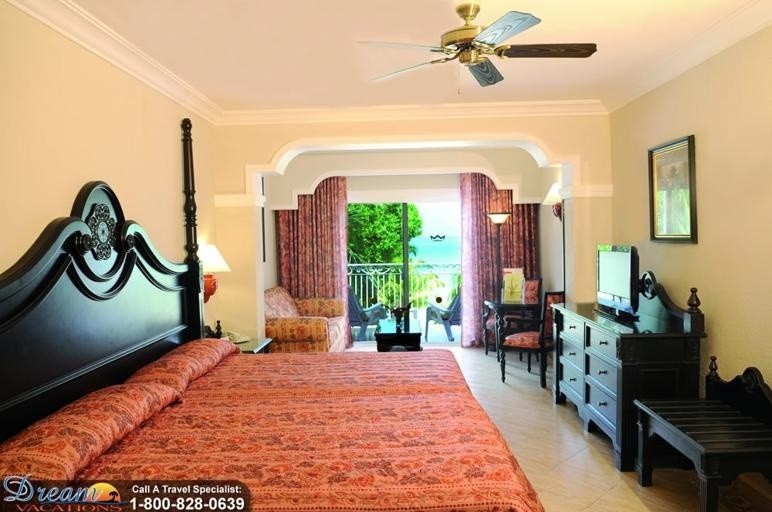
[124,337,241,397]
[1,382,183,494]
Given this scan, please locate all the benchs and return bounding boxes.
[632,356,772,512]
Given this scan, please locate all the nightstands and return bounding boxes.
[218,338,273,354]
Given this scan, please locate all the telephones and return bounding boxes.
[224,330,250,344]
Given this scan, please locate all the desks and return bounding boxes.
[482,298,540,362]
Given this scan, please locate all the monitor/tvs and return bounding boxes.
[597,244,639,321]
[592,309,636,335]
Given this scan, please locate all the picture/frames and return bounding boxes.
[647,135,698,244]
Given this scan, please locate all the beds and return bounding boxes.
[374,319,421,352]
[1,117,548,510]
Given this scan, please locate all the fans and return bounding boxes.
[373,1,597,87]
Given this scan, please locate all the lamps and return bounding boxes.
[542,182,563,204]
[196,243,232,304]
[486,213,513,305]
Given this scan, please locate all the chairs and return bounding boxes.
[498,291,565,388]
[506,278,542,334]
[264,286,346,354]
[349,286,385,341]
[425,295,462,342]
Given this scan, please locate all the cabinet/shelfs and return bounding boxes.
[550,271,708,472]
